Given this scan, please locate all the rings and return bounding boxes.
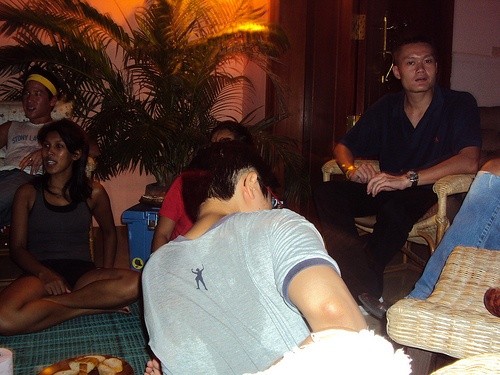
[29,157,32,161]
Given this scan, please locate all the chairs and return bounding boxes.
[386,245,500,360]
[0,105,97,266]
[321,106,499,276]
[430,352,500,374]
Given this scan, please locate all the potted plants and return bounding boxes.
[0,0,312,207]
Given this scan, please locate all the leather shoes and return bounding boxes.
[357,293,404,321]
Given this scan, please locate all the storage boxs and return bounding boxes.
[120,203,161,274]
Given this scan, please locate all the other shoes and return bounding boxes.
[351,269,383,297]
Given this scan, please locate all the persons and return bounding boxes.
[333,35,481,311]
[0,67,100,279]
[0,119,141,334]
[141,136,371,375]
[359,160,500,320]
[144,120,254,375]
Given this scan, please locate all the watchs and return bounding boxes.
[407,170,418,187]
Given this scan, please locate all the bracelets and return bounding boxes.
[345,166,356,179]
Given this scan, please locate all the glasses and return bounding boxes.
[243,169,284,208]
